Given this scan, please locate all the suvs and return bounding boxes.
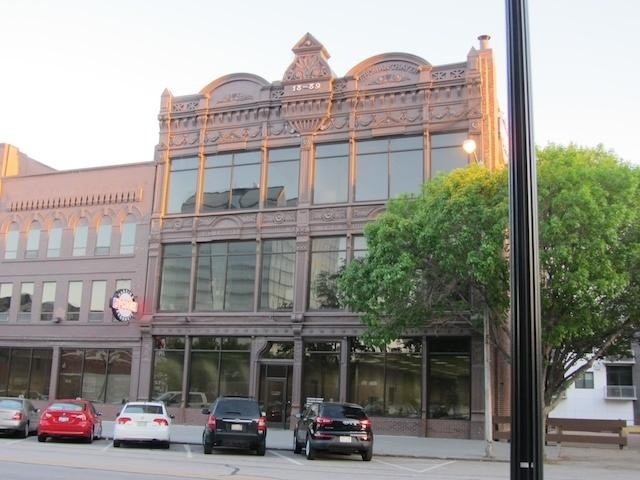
[201,396,268,457]
[292,401,374,462]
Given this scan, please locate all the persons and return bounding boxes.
[357,395,390,417]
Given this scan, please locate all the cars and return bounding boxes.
[113,401,175,449]
[360,398,457,420]
[0,397,41,438]
[34,401,103,444]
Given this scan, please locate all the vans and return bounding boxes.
[154,391,208,409]
